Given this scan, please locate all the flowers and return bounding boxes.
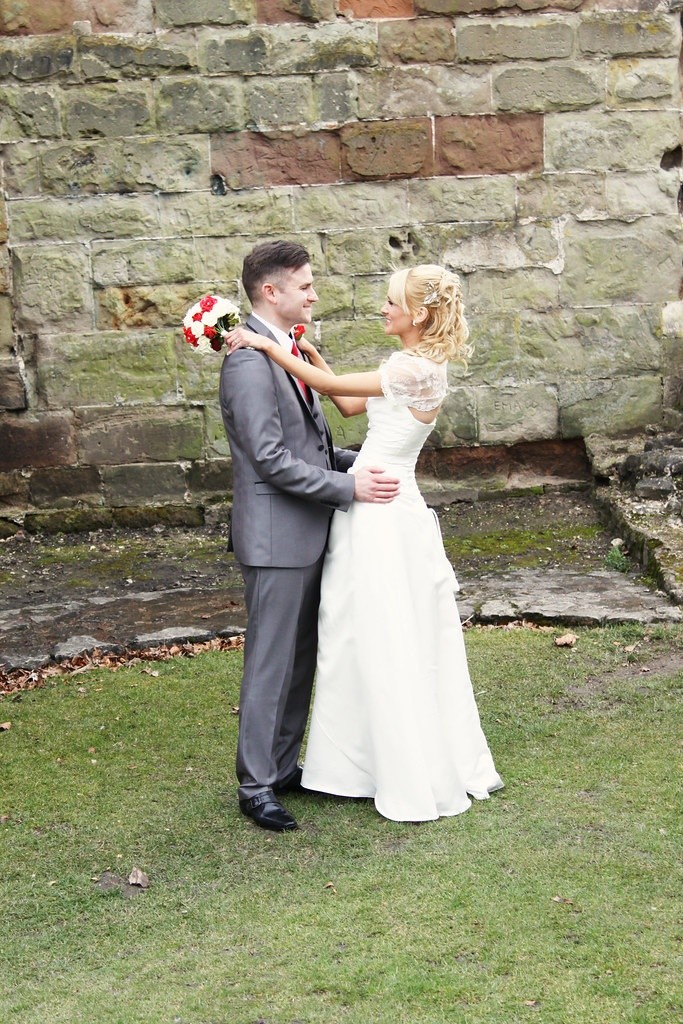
[294,323,304,339]
[181,291,241,354]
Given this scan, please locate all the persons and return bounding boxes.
[221,242,399,830]
[224,264,505,822]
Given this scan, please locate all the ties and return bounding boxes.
[291,341,308,405]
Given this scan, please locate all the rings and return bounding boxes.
[235,341,237,343]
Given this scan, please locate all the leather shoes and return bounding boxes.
[239,791,298,831]
[280,767,319,793]
